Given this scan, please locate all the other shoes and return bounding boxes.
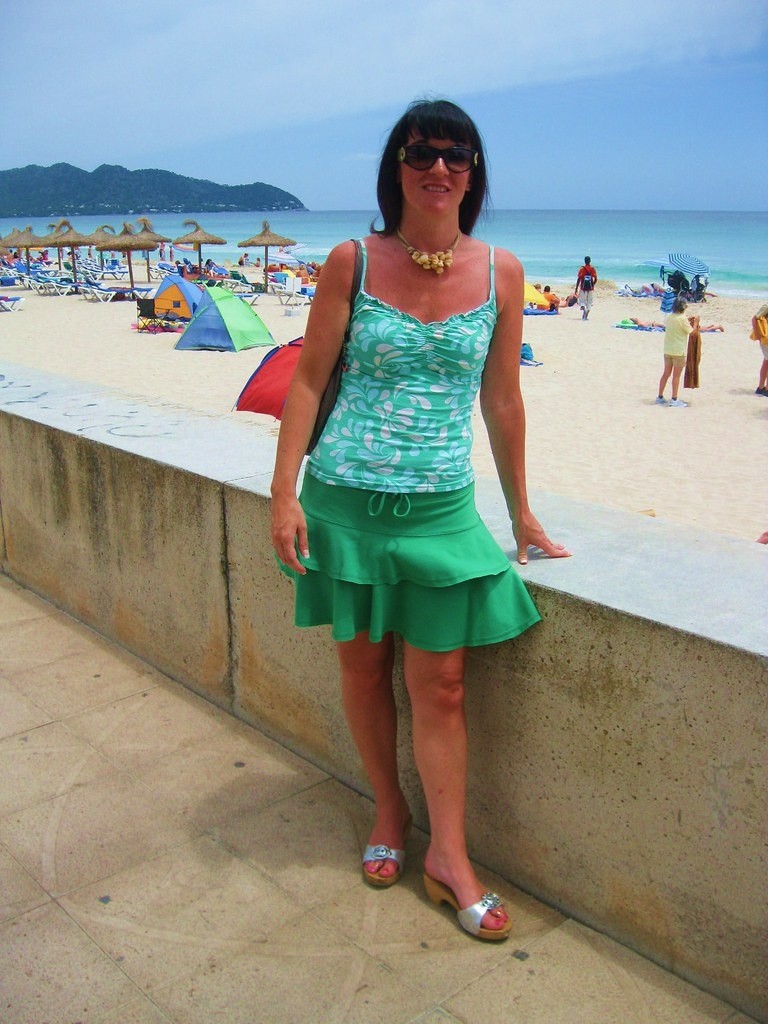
[582,309,590,319]
[755,387,768,397]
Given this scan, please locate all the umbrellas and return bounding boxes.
[283,242,307,253]
[0,218,171,289]
[262,252,299,274]
[169,242,198,253]
[670,253,711,276]
[523,280,549,307]
[238,221,297,286]
[172,219,227,272]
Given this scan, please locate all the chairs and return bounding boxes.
[0,258,156,312]
[149,262,316,307]
[136,298,173,335]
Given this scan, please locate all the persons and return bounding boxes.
[176,253,323,292]
[748,302,768,396]
[655,296,700,407]
[630,283,724,333]
[529,283,579,315]
[271,99,569,944]
[575,256,598,319]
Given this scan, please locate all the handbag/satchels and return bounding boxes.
[303,238,363,455]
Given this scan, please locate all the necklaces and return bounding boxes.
[396,227,463,276]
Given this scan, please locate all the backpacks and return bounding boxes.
[581,266,592,290]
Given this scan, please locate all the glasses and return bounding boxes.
[684,305,688,309]
[395,143,479,174]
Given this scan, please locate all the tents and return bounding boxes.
[231,335,305,422]
[173,284,279,352]
[150,273,206,323]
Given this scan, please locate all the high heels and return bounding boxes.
[423,872,512,941]
[362,813,415,886]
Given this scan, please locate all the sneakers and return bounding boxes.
[656,396,667,404]
[669,398,688,407]
